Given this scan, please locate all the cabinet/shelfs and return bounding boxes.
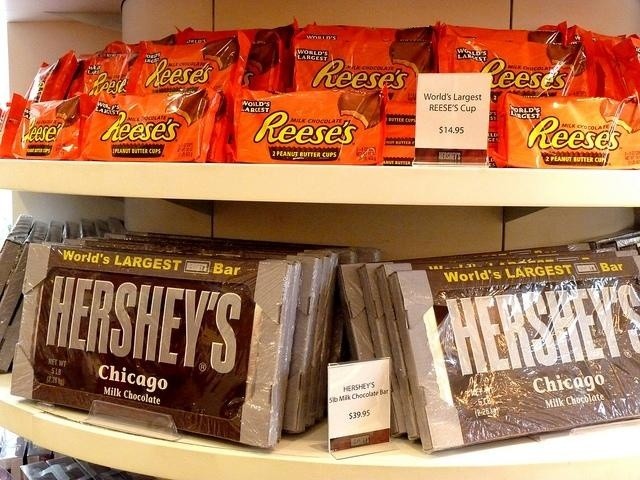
[0,1,640,480]
